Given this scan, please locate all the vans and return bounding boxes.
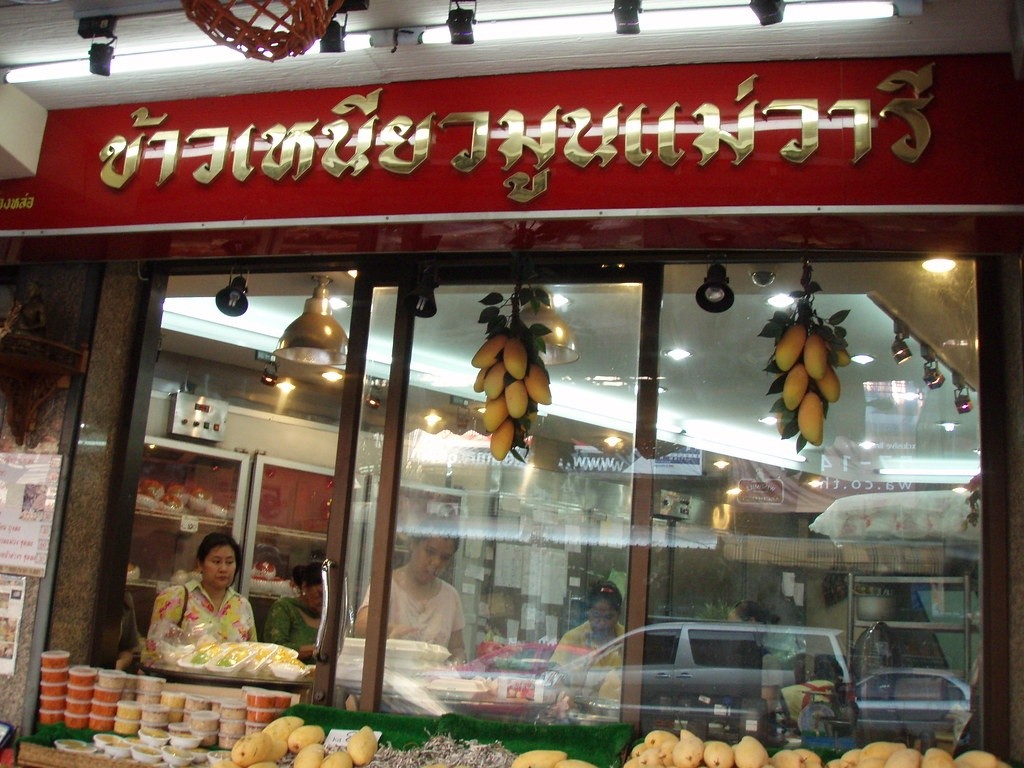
[539,622,853,744]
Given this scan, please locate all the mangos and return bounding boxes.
[776,324,851,447]
[623,729,1013,768]
[211,714,378,768]
[471,336,553,462]
[510,750,598,768]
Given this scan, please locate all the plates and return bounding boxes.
[569,714,620,725]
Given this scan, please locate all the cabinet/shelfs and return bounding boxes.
[119,435,468,664]
[843,571,983,705]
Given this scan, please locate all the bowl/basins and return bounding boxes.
[39,649,293,768]
[574,696,620,717]
[856,595,895,621]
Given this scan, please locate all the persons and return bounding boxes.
[548,581,624,707]
[141,532,257,668]
[345,517,467,711]
[728,601,783,736]
[263,561,323,665]
[484,619,504,644]
[115,536,143,670]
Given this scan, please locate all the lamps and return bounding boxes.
[272,272,349,365]
[696,254,735,313]
[891,318,913,365]
[509,282,581,366]
[215,260,251,317]
[952,369,974,415]
[920,343,945,389]
[0,0,925,87]
[403,265,442,318]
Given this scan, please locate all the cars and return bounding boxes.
[852,671,972,745]
[408,642,597,716]
